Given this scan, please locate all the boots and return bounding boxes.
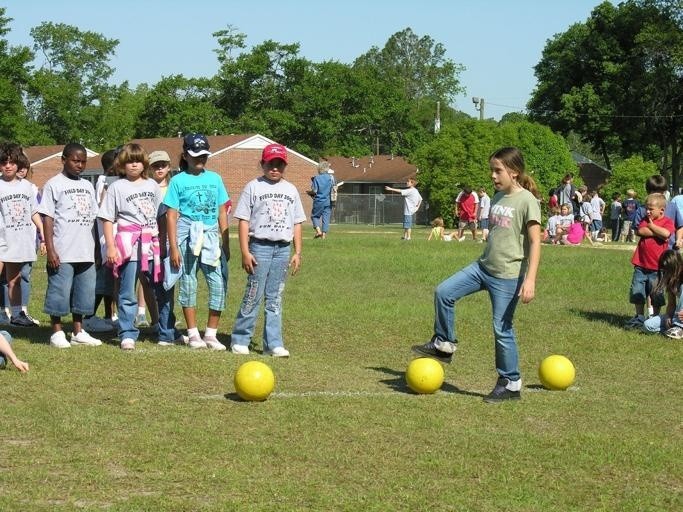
[409,337,457,366]
[481,377,523,403]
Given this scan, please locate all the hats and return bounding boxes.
[261,144,288,166]
[183,134,211,157]
[146,150,170,168]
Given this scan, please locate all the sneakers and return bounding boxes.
[261,347,290,358]
[229,340,249,356]
[48,313,226,351]
[624,314,644,329]
[0,308,40,328]
[665,326,683,339]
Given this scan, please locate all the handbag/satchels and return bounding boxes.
[330,186,337,202]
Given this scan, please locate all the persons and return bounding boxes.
[306,162,335,240]
[545,172,682,341]
[2,131,307,371]
[384,178,423,241]
[426,186,491,243]
[411,147,542,404]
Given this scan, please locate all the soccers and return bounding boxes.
[406,357,444,394]
[538,354,575,391]
[233,359,275,401]
